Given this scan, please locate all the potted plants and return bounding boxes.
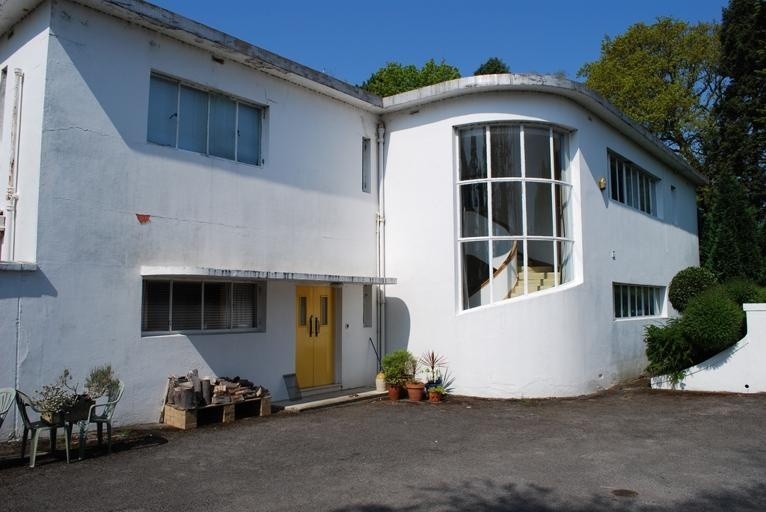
[381,349,448,404]
[33,363,119,423]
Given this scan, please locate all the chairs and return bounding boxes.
[0,378,125,469]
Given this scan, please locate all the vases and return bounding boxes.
[376,370,387,391]
